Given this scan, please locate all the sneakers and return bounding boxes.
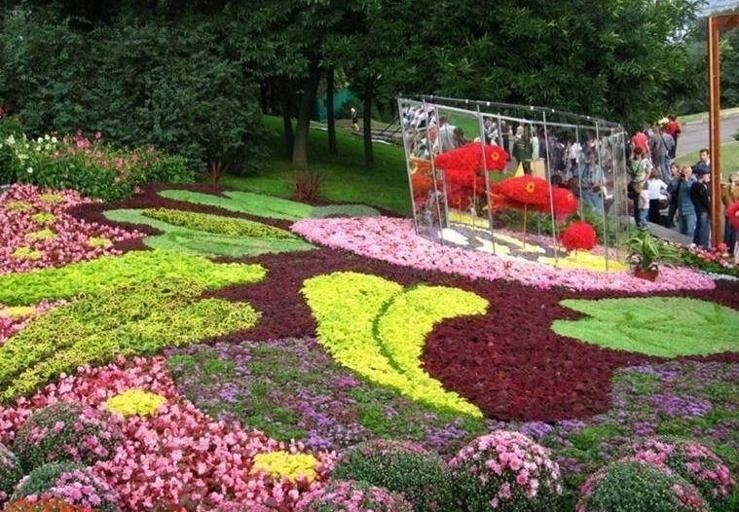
[637,223,650,231]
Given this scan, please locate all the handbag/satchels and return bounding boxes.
[658,186,672,210]
[665,149,673,160]
[627,180,649,200]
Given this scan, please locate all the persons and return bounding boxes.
[402,102,738,258]
[351,117,360,131]
[350,107,358,117]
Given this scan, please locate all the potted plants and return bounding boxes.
[621,233,684,282]
